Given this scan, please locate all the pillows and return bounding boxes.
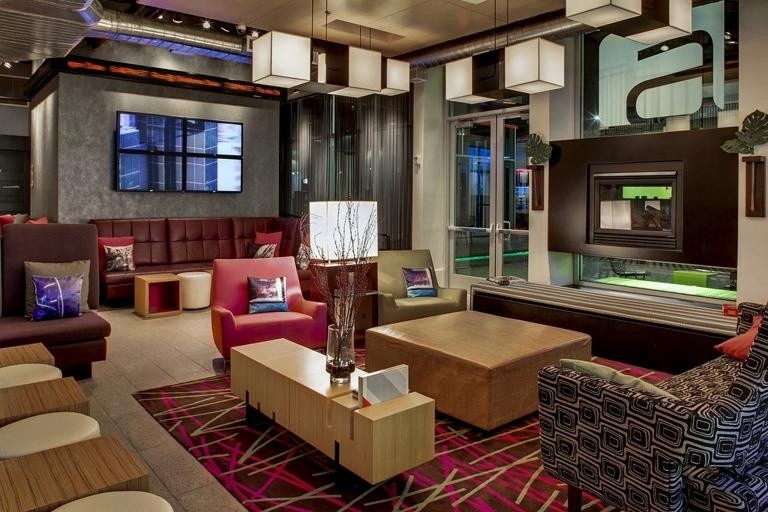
[24,260,91,318]
[560,358,681,401]
[25,217,48,224]
[32,272,84,321]
[715,317,764,362]
[14,213,29,224]
[0,215,14,231]
[247,244,278,258]
[102,245,135,272]
[255,231,282,258]
[402,266,438,297]
[99,236,135,272]
[247,276,289,314]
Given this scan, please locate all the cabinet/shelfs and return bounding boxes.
[310,262,378,340]
[470,281,738,375]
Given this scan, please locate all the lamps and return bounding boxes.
[309,200,378,264]
[504,37,564,95]
[446,57,497,104]
[252,31,311,88]
[625,0,692,46]
[375,59,410,96]
[566,0,642,28]
[317,46,382,99]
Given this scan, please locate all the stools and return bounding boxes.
[52,490,174,512]
[1,362,62,388]
[176,272,211,309]
[0,411,102,459]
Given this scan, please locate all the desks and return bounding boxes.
[231,337,435,490]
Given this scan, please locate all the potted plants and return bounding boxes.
[300,196,385,384]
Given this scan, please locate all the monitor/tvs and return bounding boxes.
[594,178,677,238]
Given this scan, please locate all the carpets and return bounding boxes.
[131,346,625,512]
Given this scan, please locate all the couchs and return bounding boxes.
[1,224,111,381]
[89,217,311,298]
[210,256,327,373]
[368,250,467,325]
[537,301,768,511]
